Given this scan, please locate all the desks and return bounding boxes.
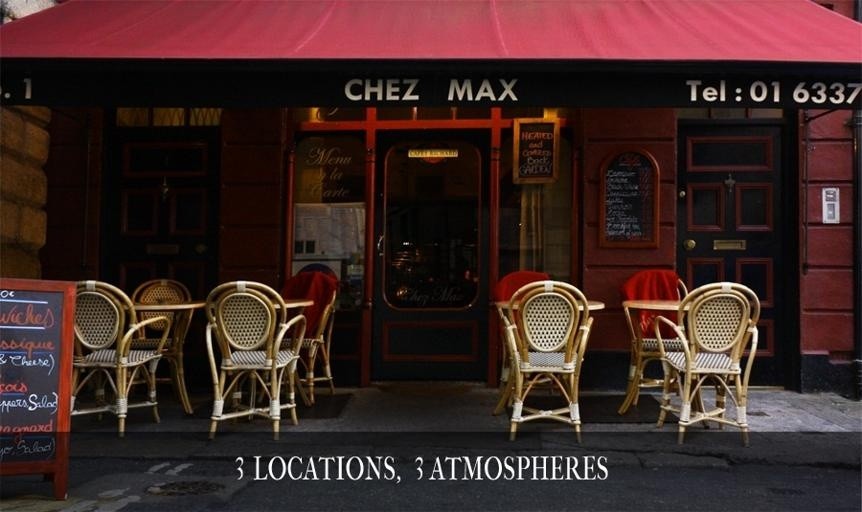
[212,300,313,422]
[618,299,711,429]
[118,299,205,416]
[494,296,605,416]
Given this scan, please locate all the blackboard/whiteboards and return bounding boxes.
[598,144,660,250]
[512,117,560,185]
[0,278,76,477]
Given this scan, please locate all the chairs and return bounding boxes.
[204,279,307,443]
[494,271,551,406]
[502,278,594,444]
[274,271,339,406]
[129,279,196,416]
[652,280,761,444]
[624,269,691,405]
[70,280,171,439]
